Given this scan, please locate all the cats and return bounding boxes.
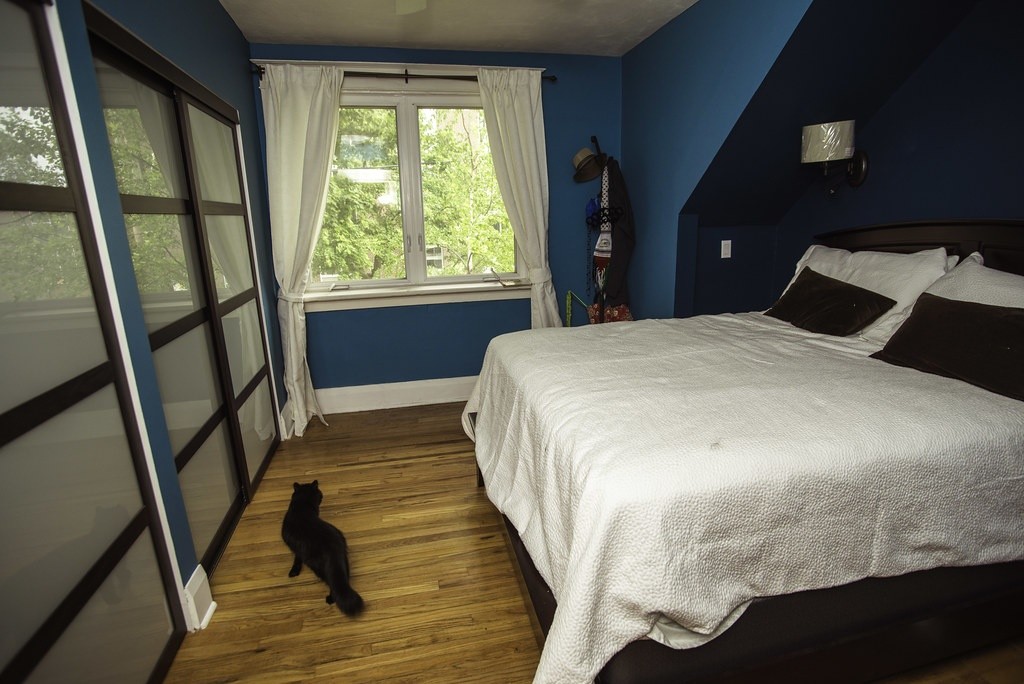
[280,480,367,619]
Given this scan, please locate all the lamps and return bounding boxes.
[800,119,869,188]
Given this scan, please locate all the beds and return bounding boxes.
[461,220,1024,684]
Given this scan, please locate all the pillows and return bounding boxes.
[763,264,897,336]
[868,292,1024,402]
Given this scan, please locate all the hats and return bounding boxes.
[573,148,607,181]
